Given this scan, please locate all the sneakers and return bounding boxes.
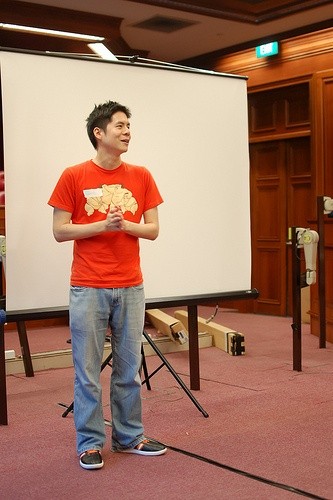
[79,449,104,469]
[112,435,167,455]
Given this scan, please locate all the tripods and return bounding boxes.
[62,316,208,420]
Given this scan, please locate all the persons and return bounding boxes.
[46,98,168,472]
